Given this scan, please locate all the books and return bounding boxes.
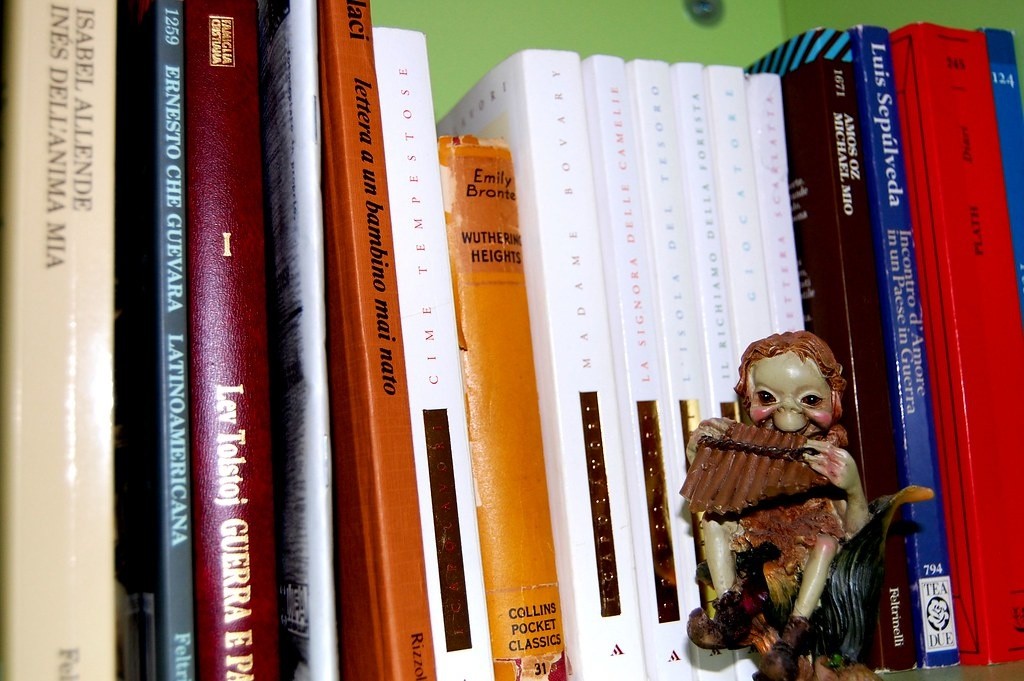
[0,0,1024,681]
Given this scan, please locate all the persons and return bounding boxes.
[682,330,884,680]
